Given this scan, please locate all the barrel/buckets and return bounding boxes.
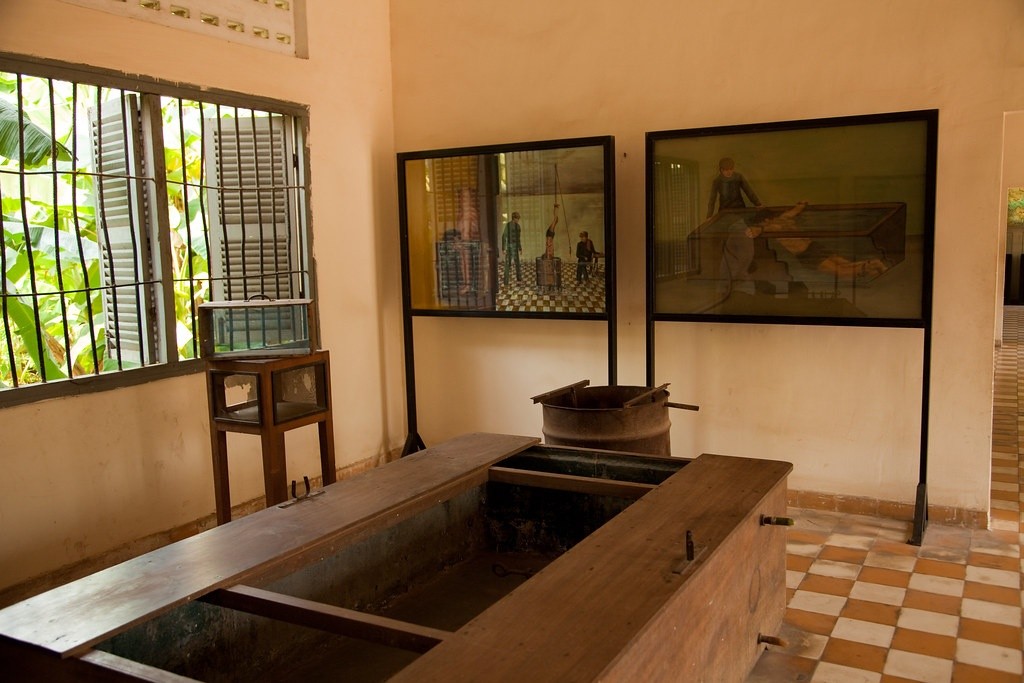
[542,386,671,456]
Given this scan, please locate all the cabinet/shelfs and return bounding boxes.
[206,349,337,525]
